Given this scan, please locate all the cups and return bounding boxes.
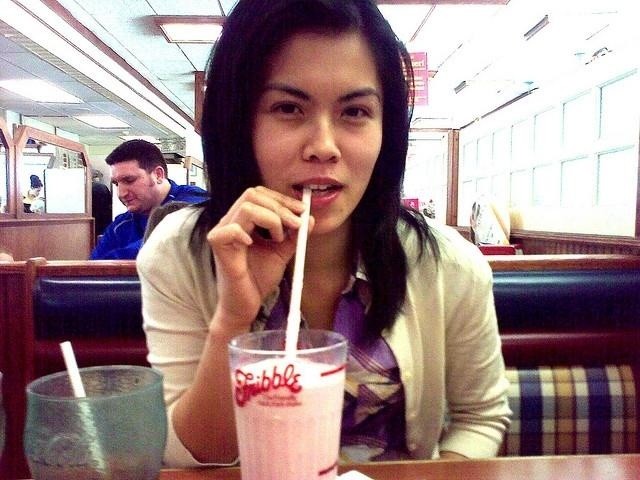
[21,363,167,480]
[226,326,350,479]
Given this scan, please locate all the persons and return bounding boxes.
[130,0,515,472]
[23,175,43,213]
[91,169,112,247]
[88,139,210,262]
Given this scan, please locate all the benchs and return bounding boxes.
[502,332,639,458]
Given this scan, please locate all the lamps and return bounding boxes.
[156,14,227,45]
